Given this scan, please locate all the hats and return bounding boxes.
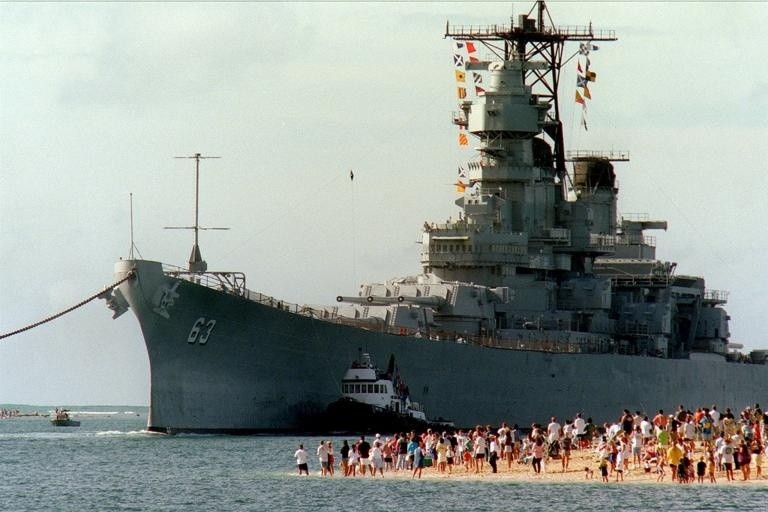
[375,433,381,438]
[427,428,432,433]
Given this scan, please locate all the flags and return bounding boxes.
[575,37,600,131]
[450,40,485,191]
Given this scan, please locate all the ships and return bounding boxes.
[93,0,768,439]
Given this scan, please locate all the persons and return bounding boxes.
[294,444,310,475]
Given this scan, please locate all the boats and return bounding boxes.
[49,407,83,427]
